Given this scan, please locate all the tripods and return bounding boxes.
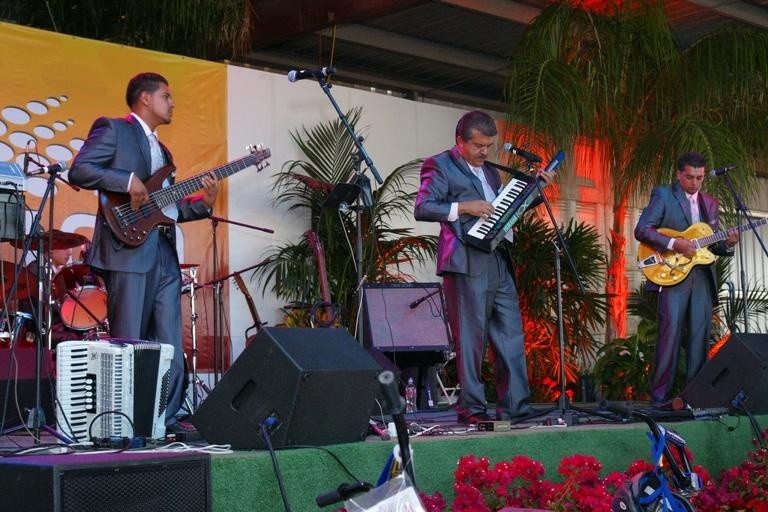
[175,268,211,432]
[511,170,626,426]
[1,176,76,448]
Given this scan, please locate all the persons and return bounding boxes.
[633,150,741,409]
[67,71,221,442]
[413,109,550,425]
[19,228,82,348]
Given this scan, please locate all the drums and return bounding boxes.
[51,267,108,330]
[0,311,37,351]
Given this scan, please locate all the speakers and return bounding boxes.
[682,332,764,416]
[192,324,386,452]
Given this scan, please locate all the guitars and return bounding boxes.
[101,144,271,246]
[638,219,768,287]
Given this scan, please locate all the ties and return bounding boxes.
[689,197,699,225]
[474,167,515,244]
[147,134,179,222]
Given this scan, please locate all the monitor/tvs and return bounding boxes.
[364,281,455,354]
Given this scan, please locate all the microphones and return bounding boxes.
[409,291,443,309]
[504,141,544,164]
[23,146,30,173]
[29,159,71,177]
[287,63,337,82]
[711,164,741,177]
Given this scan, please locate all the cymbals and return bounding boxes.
[0,261,37,300]
[10,232,87,251]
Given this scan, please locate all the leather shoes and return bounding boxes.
[457,409,493,427]
[165,419,195,433]
[496,404,557,425]
